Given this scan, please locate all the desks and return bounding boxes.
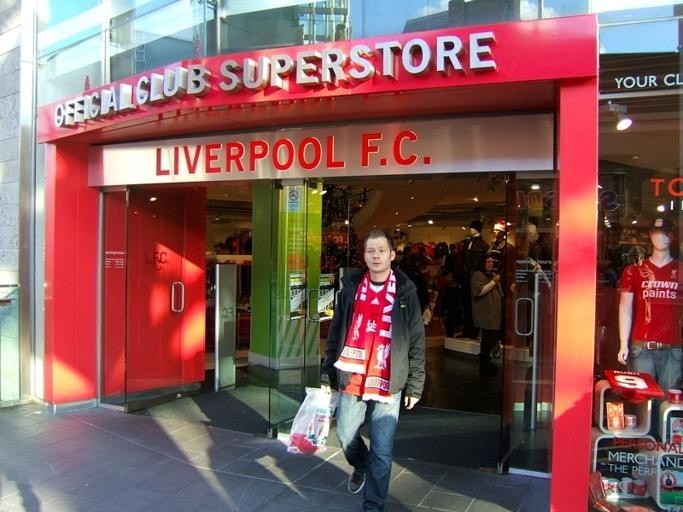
[205,308,252,345]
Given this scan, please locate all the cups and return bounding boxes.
[668,389,681,403]
[602,477,647,496]
[624,415,637,429]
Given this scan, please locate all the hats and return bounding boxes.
[493,218,506,233]
[647,216,675,230]
[470,220,482,232]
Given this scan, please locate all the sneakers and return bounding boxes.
[348,472,366,493]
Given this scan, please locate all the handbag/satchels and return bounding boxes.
[286,385,339,455]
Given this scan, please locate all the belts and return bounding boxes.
[632,341,683,350]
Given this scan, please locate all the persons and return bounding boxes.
[455,217,488,339]
[614,210,682,438]
[320,230,428,511]
[487,218,514,296]
[209,234,501,374]
[509,215,552,351]
